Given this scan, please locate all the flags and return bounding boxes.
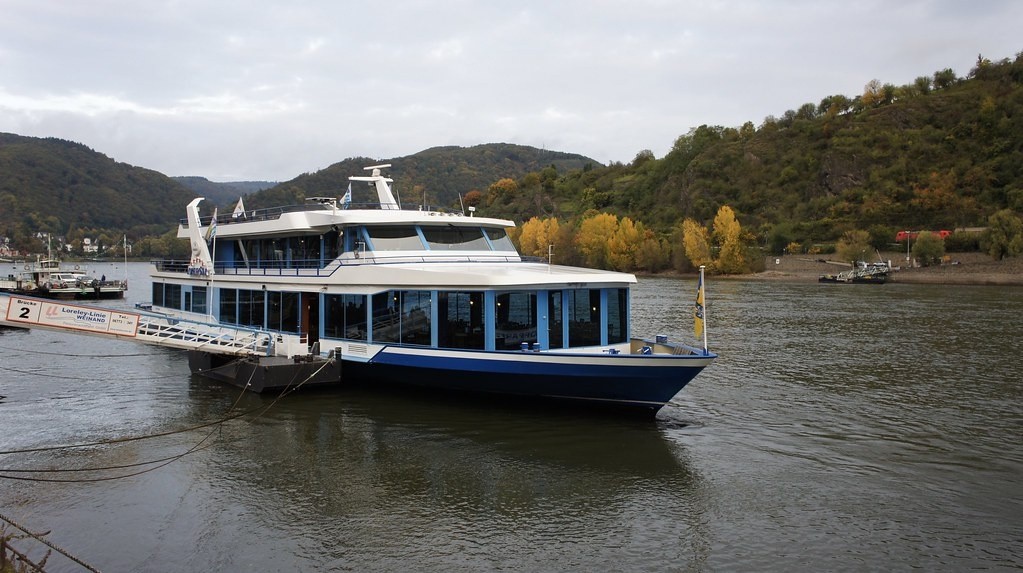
[693,272,703,340]
[204,210,216,246]
[232,199,244,217]
[339,182,351,210]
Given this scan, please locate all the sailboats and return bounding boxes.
[0,230,129,301]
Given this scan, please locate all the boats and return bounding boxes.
[818,260,890,285]
[0,161,718,424]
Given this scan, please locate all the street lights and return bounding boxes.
[904,229,911,264]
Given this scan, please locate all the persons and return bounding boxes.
[851,256,859,270]
